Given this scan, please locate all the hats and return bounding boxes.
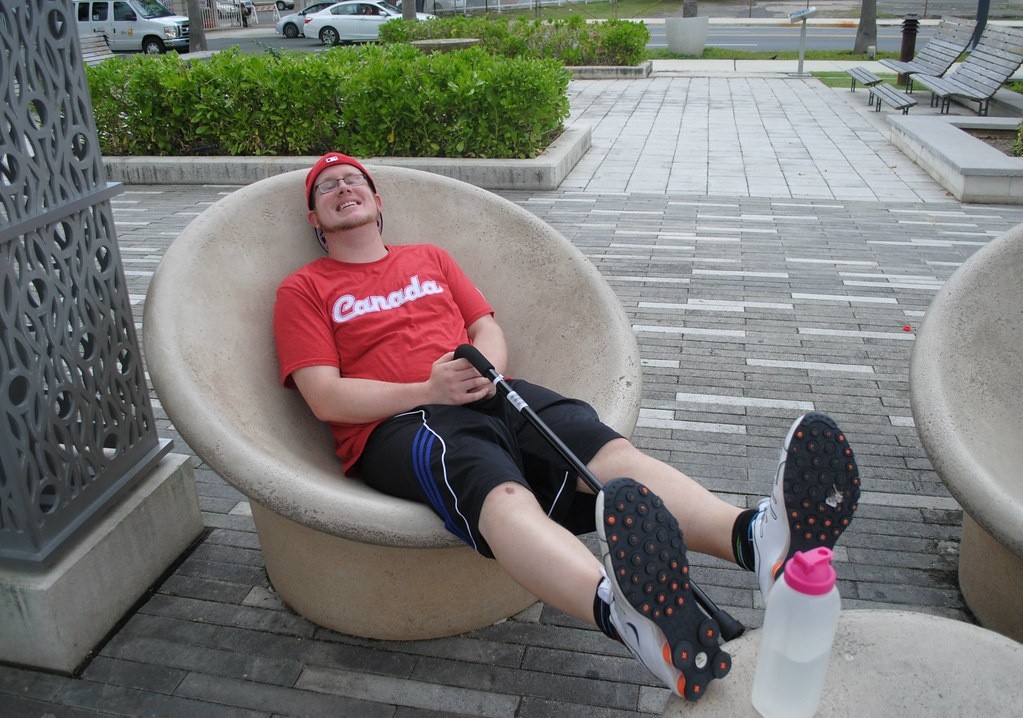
[305,153,383,253]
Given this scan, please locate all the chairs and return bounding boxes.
[144,164,644,641]
[909,218,1023,644]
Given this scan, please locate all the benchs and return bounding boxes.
[846,65,883,105]
[180,50,222,61]
[877,15,978,104]
[5,32,124,94]
[872,82,917,114]
[908,24,1023,116]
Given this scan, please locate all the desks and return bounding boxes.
[662,609,1023,718]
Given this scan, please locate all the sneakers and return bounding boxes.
[752,412,863,606]
[595,478,732,704]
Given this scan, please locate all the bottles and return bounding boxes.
[751,546,841,718]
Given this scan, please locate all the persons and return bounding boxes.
[274,153,863,702]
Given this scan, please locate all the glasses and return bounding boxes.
[313,174,368,194]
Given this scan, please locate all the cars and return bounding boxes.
[253,0,295,11]
[303,0,440,48]
[214,0,239,20]
[276,0,356,41]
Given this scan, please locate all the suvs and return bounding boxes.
[54,0,189,58]
